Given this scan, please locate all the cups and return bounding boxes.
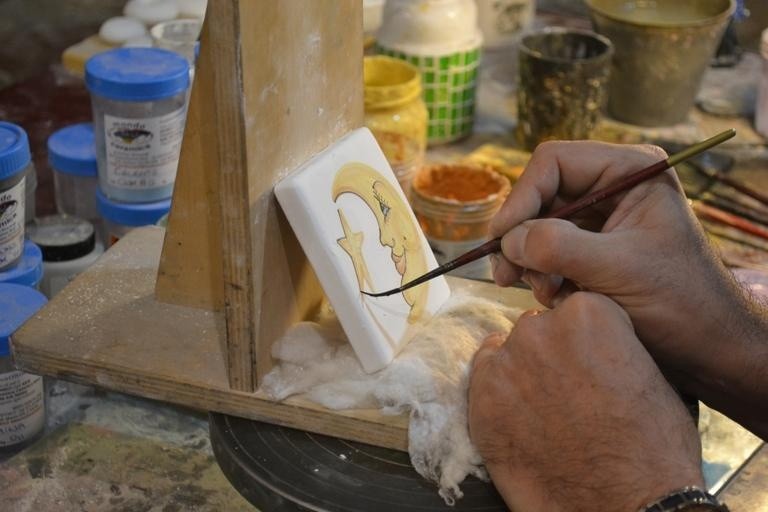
[149,18,203,67]
[514,29,617,155]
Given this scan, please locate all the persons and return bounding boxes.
[467,140,768,512]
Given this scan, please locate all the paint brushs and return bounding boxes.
[361,128,735,296]
[686,197,768,245]
[698,218,768,260]
[687,159,768,203]
[677,180,768,227]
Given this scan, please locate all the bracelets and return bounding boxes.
[638,486,729,512]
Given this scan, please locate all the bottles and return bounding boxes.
[402,166,516,280]
[1,121,32,273]
[94,182,172,252]
[46,123,99,224]
[357,57,432,189]
[0,282,50,461]
[753,27,768,138]
[24,214,105,299]
[25,157,39,224]
[85,47,191,204]
[0,238,44,293]
